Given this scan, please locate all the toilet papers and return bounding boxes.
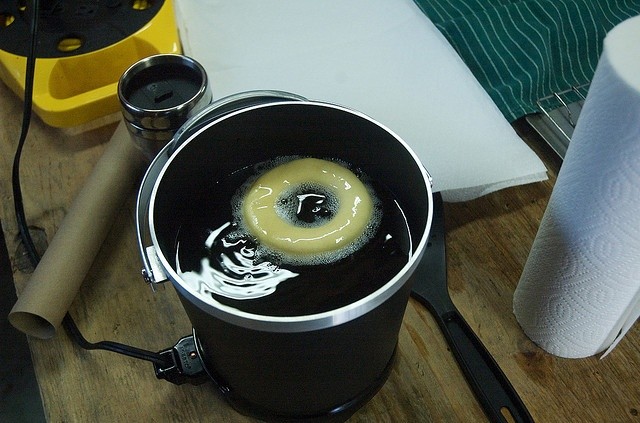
[512,15,640,361]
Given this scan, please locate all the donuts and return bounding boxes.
[240,158,374,259]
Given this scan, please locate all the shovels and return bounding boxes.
[410,192,535,423]
